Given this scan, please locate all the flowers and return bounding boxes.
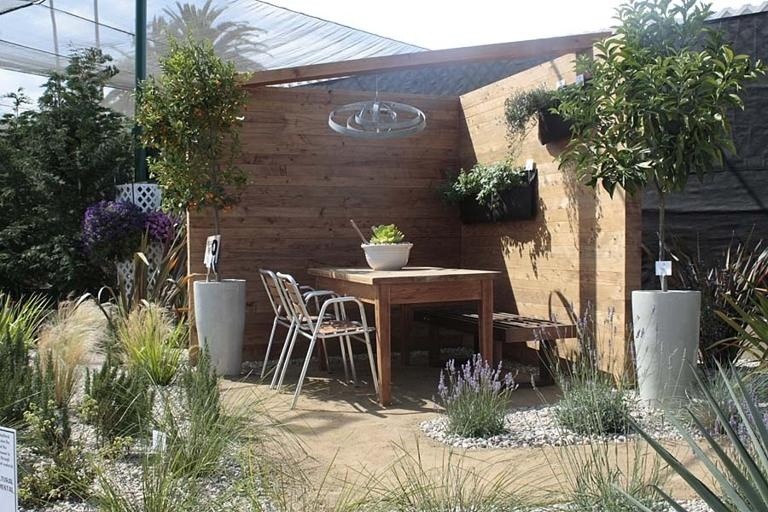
[78,197,177,265]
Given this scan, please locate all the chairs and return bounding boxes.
[258,268,350,390]
[276,271,378,410]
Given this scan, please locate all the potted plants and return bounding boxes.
[436,160,538,225]
[360,223,414,270]
[547,0,767,401]
[496,83,585,168]
[132,33,257,377]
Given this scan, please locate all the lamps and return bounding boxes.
[328,74,427,139]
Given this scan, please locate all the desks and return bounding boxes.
[307,265,502,407]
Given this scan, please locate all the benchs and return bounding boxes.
[413,307,593,390]
